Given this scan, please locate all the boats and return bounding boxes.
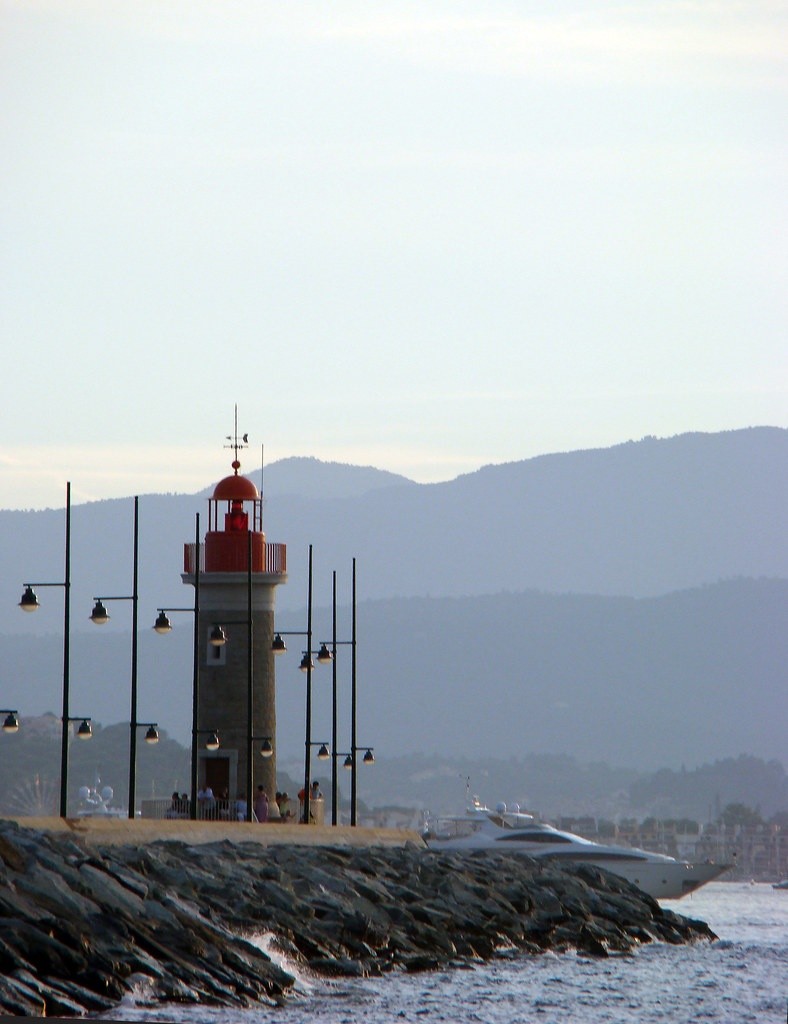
[416,800,736,900]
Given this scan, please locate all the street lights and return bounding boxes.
[89,495,161,817]
[1,478,91,818]
[272,546,330,823]
[155,513,220,819]
[319,556,377,827]
[212,530,273,824]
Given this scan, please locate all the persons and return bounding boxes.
[253,785,269,823]
[298,781,322,819]
[165,792,190,820]
[197,783,247,821]
[276,792,291,817]
